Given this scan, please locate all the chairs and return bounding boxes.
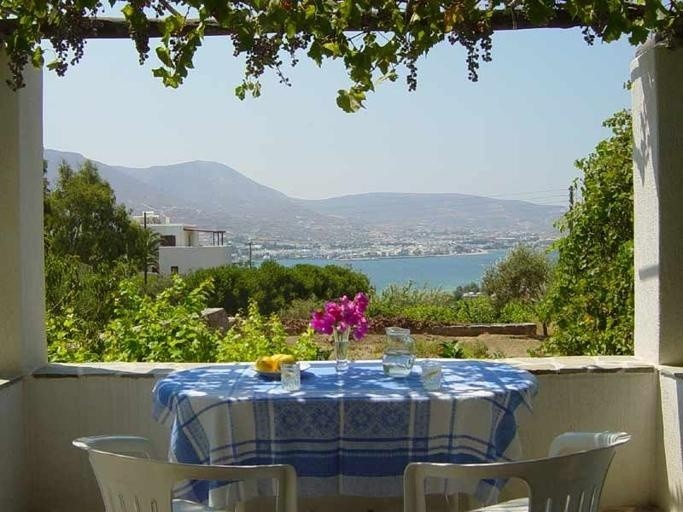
[68,433,298,511]
[398,428,634,511]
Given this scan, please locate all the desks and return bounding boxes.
[159,357,537,510]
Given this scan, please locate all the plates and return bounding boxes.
[250,363,311,377]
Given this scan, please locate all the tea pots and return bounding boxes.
[383,327,415,379]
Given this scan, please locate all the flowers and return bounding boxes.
[309,289,370,358]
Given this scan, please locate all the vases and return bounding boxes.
[330,326,351,374]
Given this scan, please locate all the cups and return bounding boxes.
[279,363,301,391]
[421,361,441,391]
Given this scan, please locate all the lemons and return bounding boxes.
[256,353,295,370]
